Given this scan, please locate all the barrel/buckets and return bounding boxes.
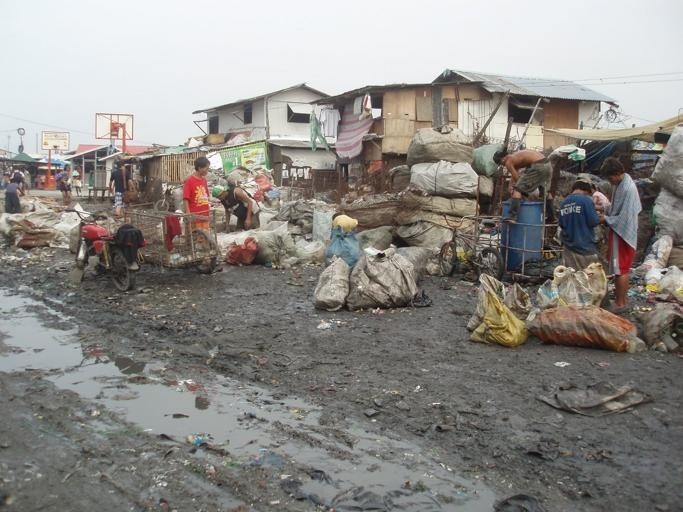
[499,200,545,272]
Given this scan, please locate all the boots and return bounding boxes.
[505,198,520,220]
[546,199,554,224]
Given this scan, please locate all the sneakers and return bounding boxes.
[608,304,627,315]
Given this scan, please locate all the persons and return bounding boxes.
[183,156,221,241]
[212,188,261,233]
[559,180,611,309]
[1,167,32,212]
[493,151,553,224]
[56,164,71,202]
[72,165,82,196]
[88,166,95,185]
[598,156,643,312]
[590,183,611,215]
[109,161,131,215]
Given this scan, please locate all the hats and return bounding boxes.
[493,148,507,164]
[212,186,229,197]
[577,173,597,191]
